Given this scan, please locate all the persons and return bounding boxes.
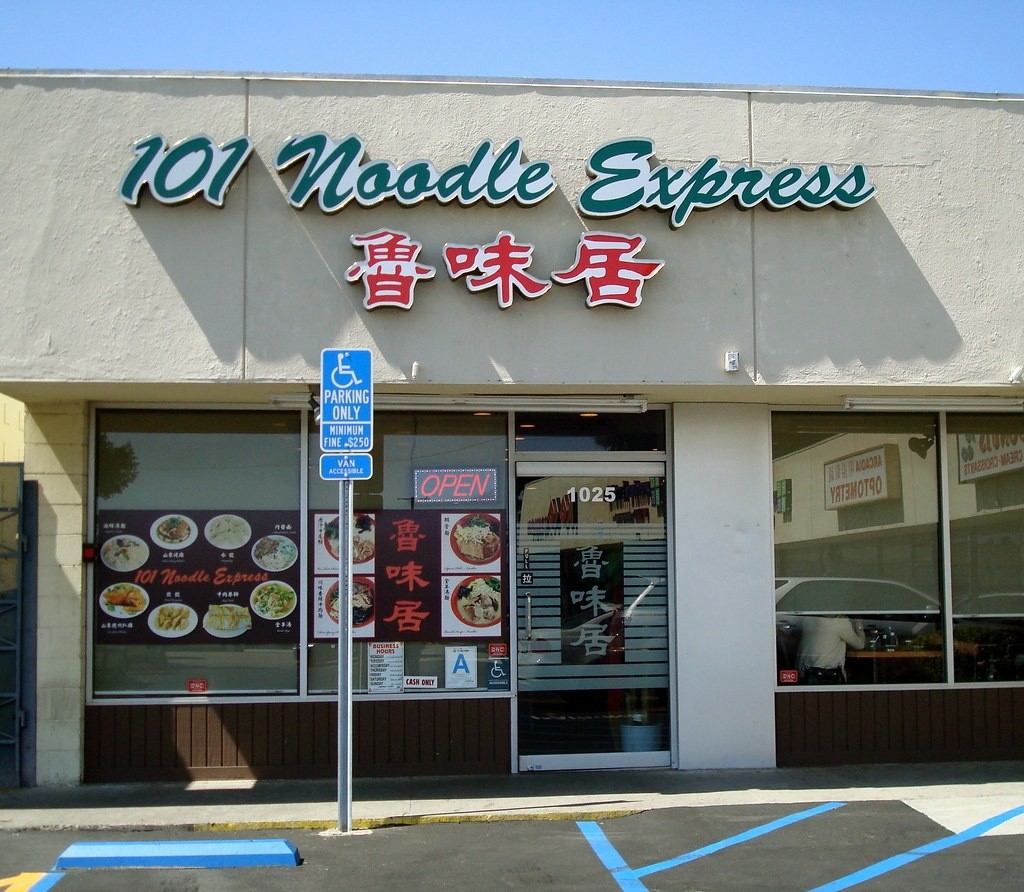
[924,621,992,682]
[794,613,865,685]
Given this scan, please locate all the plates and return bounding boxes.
[251,535,298,572]
[324,576,375,628]
[204,514,252,550]
[99,582,149,618]
[250,580,298,621]
[449,514,502,566]
[324,513,375,564]
[148,603,197,638]
[100,534,149,572]
[451,575,502,627]
[203,603,249,638]
[150,514,198,550]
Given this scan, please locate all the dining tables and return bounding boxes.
[846,646,946,685]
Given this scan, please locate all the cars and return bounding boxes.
[915,591,1023,639]
[776,577,942,641]
